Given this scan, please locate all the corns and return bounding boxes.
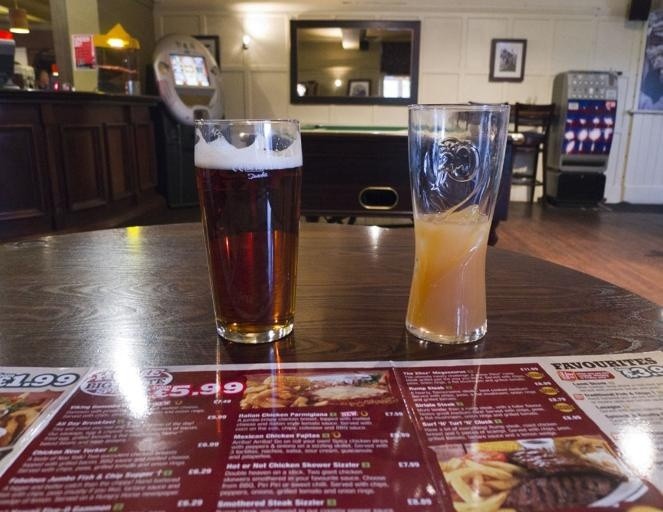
[264,376,311,388]
[476,441,520,451]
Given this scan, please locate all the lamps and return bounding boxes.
[8,0,30,34]
[339,28,362,51]
[242,34,250,49]
[92,22,141,51]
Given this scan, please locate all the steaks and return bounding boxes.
[503,473,618,512]
[508,448,628,481]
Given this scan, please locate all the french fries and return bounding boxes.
[439,457,524,512]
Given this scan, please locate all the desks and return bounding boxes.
[238,125,512,247]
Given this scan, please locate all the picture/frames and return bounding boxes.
[489,38,527,82]
[347,80,371,98]
[193,35,220,70]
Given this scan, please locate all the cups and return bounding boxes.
[403,101,512,347]
[192,116,306,346]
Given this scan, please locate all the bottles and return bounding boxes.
[49,64,61,90]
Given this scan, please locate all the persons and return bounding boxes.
[35,70,50,91]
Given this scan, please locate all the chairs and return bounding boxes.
[511,101,555,204]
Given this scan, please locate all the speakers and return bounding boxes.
[629,0,652,21]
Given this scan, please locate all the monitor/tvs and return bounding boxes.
[168,53,211,87]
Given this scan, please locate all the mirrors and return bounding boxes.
[289,19,421,106]
